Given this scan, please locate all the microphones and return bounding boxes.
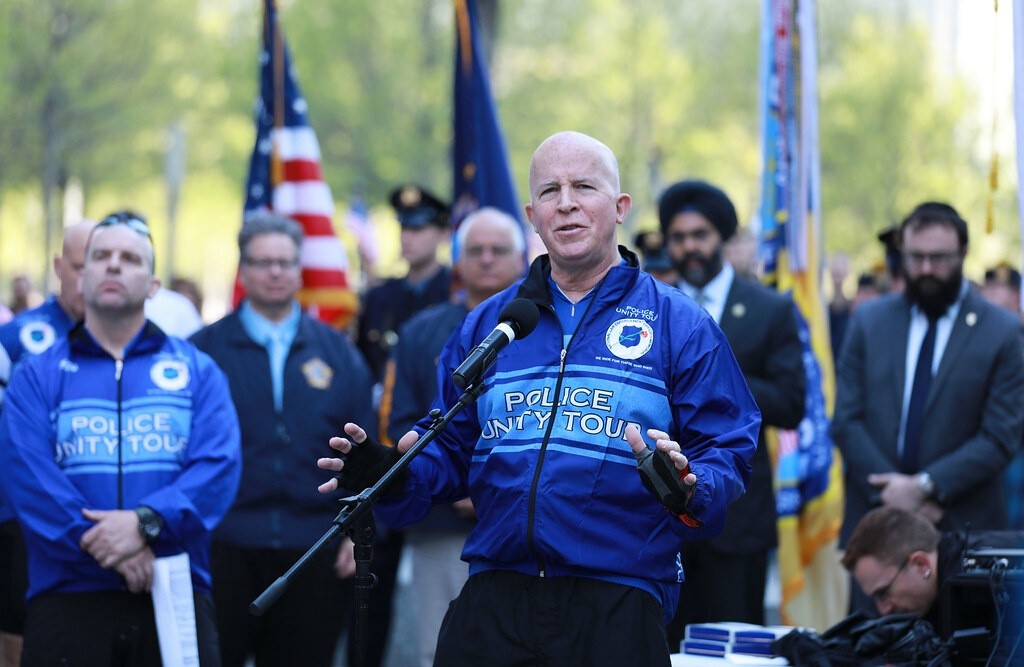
[452,297,540,389]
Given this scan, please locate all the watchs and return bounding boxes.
[135,506,165,540]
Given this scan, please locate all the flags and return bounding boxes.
[441,0,531,287]
[235,1,360,329]
[760,1,844,614]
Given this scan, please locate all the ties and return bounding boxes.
[268,325,289,411]
[902,304,945,458]
[693,292,709,306]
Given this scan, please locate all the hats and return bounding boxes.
[655,178,738,245]
[985,264,1020,287]
[390,184,455,230]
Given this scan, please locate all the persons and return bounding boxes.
[185,213,377,667]
[0,209,244,667]
[364,92,812,667]
[826,200,1024,667]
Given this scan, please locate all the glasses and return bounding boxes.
[100,214,150,236]
[904,250,958,271]
[242,256,301,272]
[868,558,909,607]
